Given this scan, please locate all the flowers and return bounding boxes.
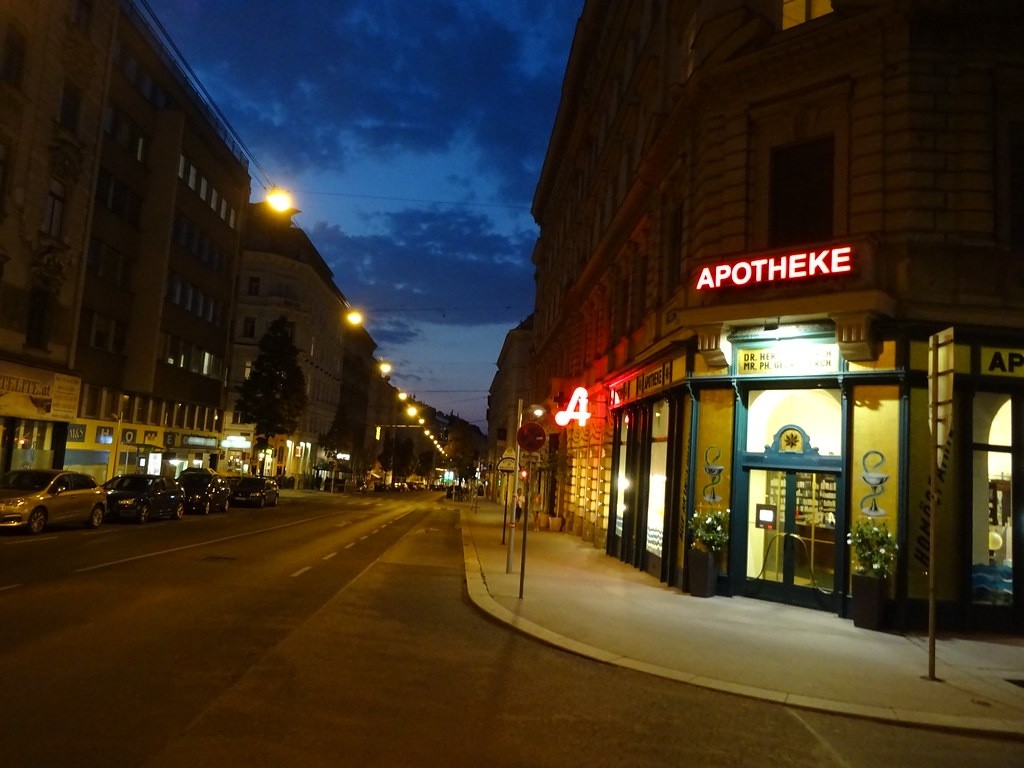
[847,517,899,578]
[687,508,730,552]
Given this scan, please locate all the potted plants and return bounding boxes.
[538,450,571,531]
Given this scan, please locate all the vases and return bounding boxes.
[852,574,886,630]
[688,549,719,597]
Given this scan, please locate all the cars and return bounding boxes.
[229,476,279,509]
[362,481,449,497]
[447,486,463,498]
[0,468,108,536]
[101,473,187,525]
[176,469,232,515]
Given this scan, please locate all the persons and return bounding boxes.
[513,487,542,532]
